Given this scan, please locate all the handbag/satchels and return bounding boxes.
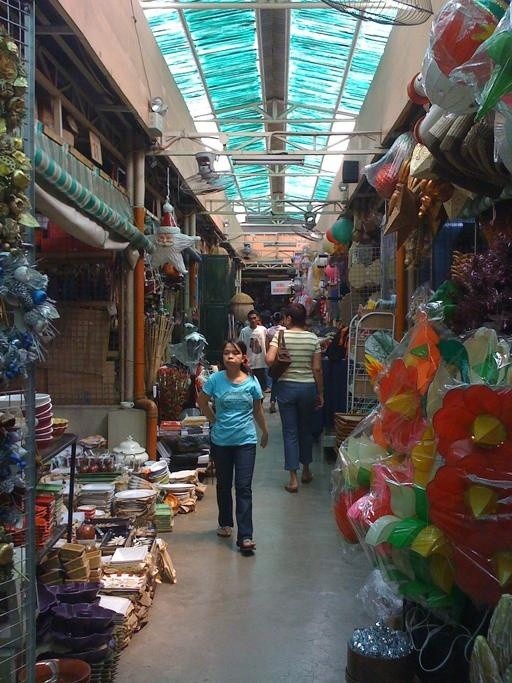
[268,347,292,381]
[326,343,346,361]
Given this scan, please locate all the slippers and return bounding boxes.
[301,472,313,483]
[217,523,231,537]
[285,483,297,492]
[241,541,256,551]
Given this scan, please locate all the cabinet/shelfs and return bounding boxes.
[344,311,395,413]
[1,429,74,589]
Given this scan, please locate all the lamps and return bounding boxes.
[148,97,164,113]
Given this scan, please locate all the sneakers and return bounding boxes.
[270,403,276,413]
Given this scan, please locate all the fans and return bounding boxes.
[179,153,237,197]
[288,211,325,243]
[233,243,262,262]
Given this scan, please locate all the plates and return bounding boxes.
[58,434,115,461]
[5,483,62,549]
[109,544,148,565]
[76,482,115,518]
[159,465,198,500]
[115,488,157,498]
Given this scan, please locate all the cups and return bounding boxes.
[121,400,134,409]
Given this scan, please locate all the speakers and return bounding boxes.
[341,159,360,184]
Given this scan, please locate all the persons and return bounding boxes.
[197,338,270,551]
[235,307,288,414]
[263,302,324,492]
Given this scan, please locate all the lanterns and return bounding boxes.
[428,0,511,89]
[325,226,340,244]
[332,217,354,245]
[420,46,484,115]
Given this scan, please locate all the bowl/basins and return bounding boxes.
[77,504,95,517]
[52,418,69,436]
[0,392,54,451]
[36,580,119,682]
[36,542,103,584]
[129,459,170,487]
